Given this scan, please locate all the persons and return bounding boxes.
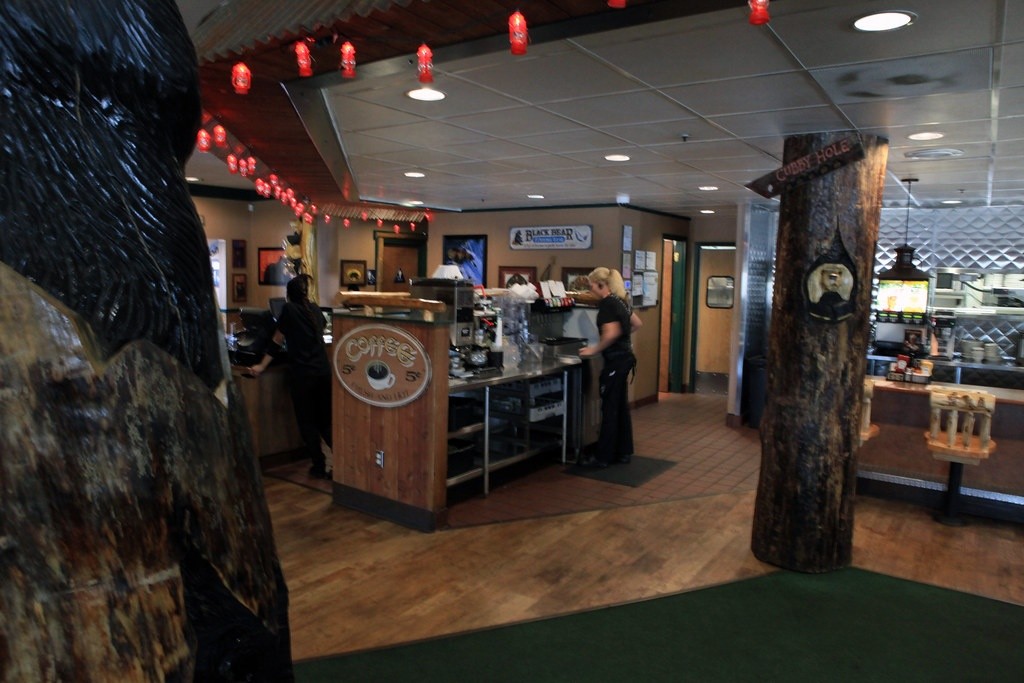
[247,274,332,479]
[578,266,643,468]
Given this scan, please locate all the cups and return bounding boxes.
[365,360,395,390]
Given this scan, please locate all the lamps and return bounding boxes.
[878,179,936,314]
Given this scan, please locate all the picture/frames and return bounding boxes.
[231,239,247,303]
[258,246,286,286]
[560,266,598,307]
[340,260,368,287]
[499,265,537,289]
[442,234,488,323]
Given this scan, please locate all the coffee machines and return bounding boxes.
[411,278,474,376]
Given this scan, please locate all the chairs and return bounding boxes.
[923,384,996,527]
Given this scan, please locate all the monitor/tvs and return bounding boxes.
[239,308,283,351]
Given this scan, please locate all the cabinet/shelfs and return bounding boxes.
[867,305,1023,373]
[856,372,1024,498]
[446,354,584,496]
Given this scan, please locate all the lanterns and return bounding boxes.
[233,62,251,94]
[196,123,435,236]
[339,40,354,76]
[295,42,313,75]
[416,44,433,85]
[748,1,770,23]
[508,11,528,56]
[606,0,625,6]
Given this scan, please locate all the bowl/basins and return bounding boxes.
[961,338,1003,362]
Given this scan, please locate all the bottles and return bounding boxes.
[229,323,238,351]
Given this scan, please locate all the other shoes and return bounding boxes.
[612,455,631,464]
[309,465,326,478]
[588,459,609,471]
